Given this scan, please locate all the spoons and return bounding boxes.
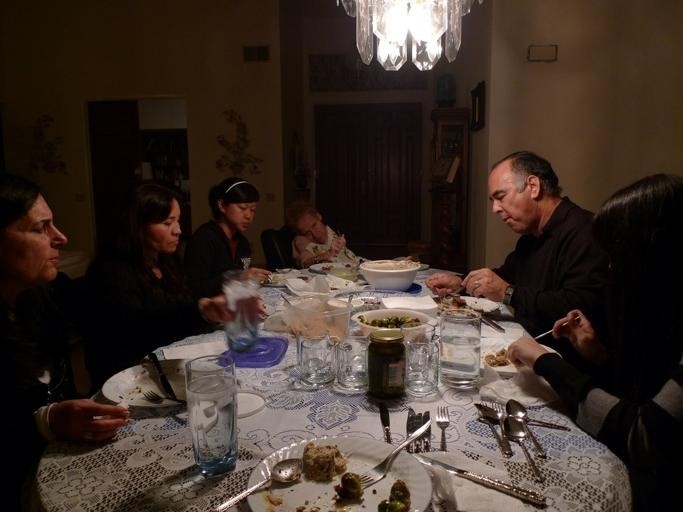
[213,454,304,511]
[476,401,571,480]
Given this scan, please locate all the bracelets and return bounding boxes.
[502,283,515,306]
[35,403,58,445]
[326,248,333,259]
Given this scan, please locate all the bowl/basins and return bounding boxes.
[351,307,437,340]
[361,258,419,290]
[335,290,412,309]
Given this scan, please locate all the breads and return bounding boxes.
[301,442,347,480]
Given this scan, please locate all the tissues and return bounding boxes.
[379,295,438,322]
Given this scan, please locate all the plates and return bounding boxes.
[247,436,433,512]
[431,294,501,319]
[484,343,559,375]
[103,357,224,415]
[264,273,310,287]
[312,259,360,275]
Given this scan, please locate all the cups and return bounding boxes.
[183,353,238,474]
[220,271,262,352]
[287,312,482,397]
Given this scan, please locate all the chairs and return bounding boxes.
[259,228,290,269]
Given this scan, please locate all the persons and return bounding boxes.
[282,198,360,269]
[90,178,269,372]
[501,173,683,512]
[173,177,275,298]
[1,172,130,511]
[424,150,599,359]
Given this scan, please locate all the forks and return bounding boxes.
[360,414,431,488]
[479,401,512,457]
[435,406,451,452]
[143,385,186,406]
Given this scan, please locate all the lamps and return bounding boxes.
[336,0,474,76]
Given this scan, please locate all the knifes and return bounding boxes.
[149,351,179,400]
[406,453,548,507]
[377,404,433,452]
[461,303,504,333]
